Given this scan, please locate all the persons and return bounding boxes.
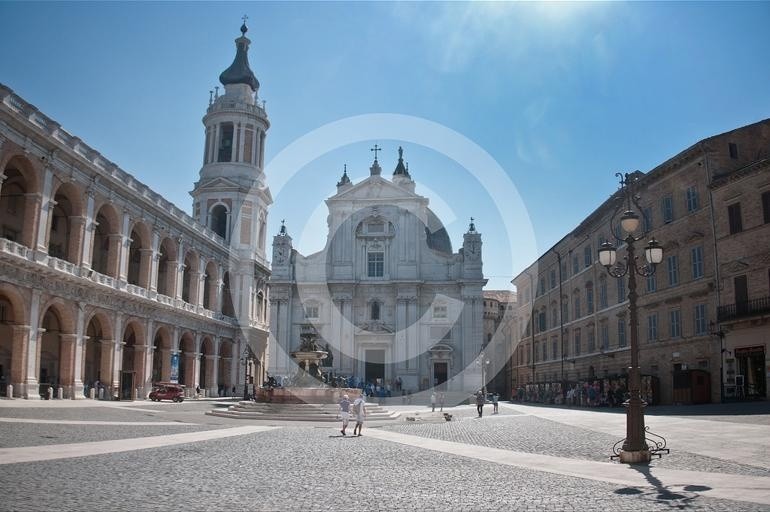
[349,376,402,398]
[232,383,236,397]
[336,395,352,435]
[94,380,100,398]
[354,394,367,436]
[402,388,406,404]
[407,390,413,403]
[477,391,484,417]
[431,392,437,412]
[492,391,500,414]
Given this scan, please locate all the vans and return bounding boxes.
[149,385,184,402]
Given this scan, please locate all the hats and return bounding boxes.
[343,395,349,399]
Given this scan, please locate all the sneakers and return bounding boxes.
[358,434,361,436]
[354,429,356,435]
[341,430,346,435]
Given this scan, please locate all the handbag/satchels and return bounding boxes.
[353,404,360,413]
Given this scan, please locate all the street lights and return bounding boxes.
[240,343,260,400]
[476,350,489,403]
[599,169,668,464]
